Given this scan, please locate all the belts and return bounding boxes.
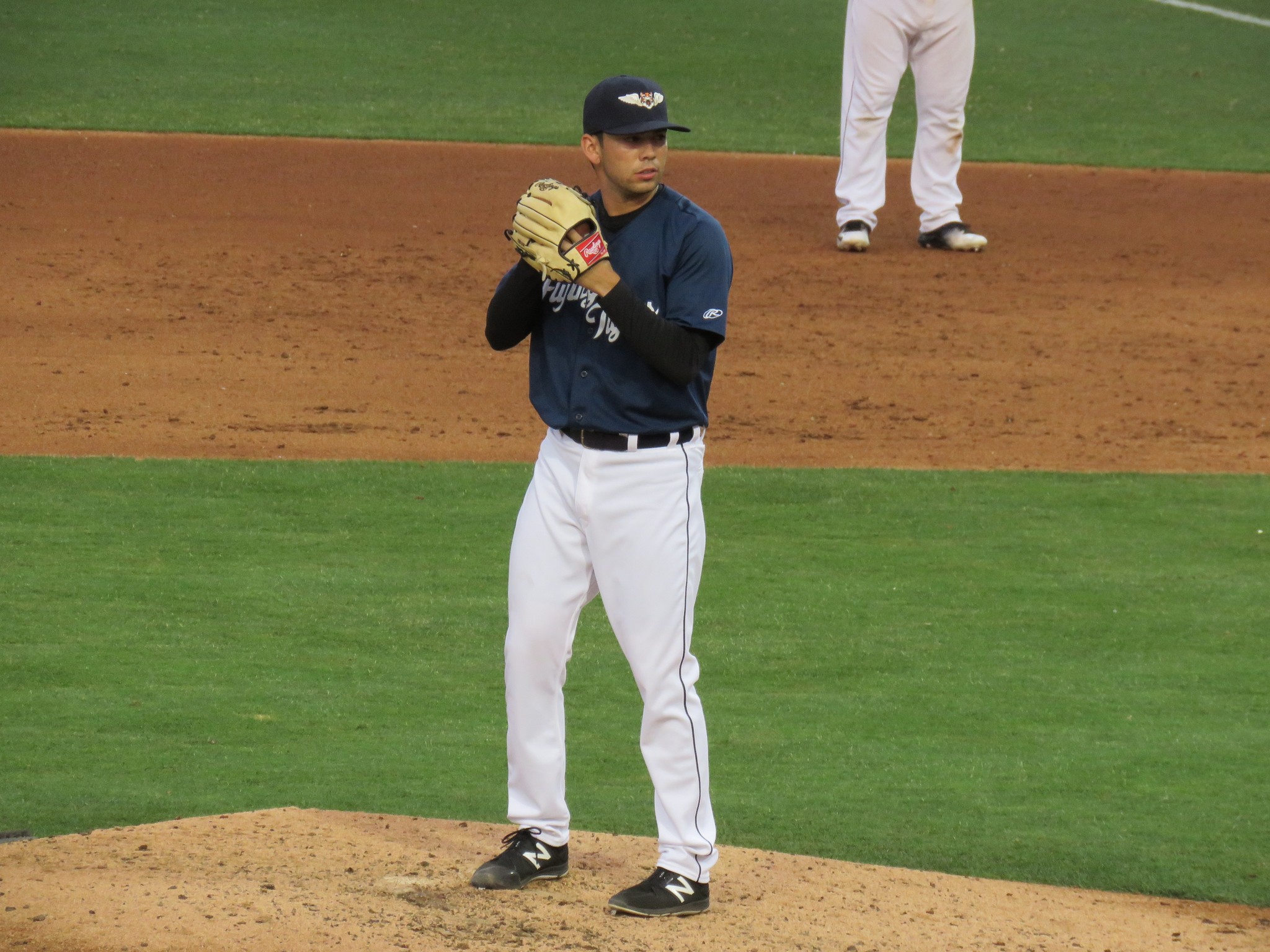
[557,422,695,452]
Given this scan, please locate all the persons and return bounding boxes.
[836,1,989,251]
[469,75,732,920]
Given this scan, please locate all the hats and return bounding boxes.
[582,75,691,135]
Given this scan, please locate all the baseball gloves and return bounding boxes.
[510,179,610,288]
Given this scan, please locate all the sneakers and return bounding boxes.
[917,222,987,251]
[836,220,871,251]
[607,865,711,917]
[470,826,570,890]
